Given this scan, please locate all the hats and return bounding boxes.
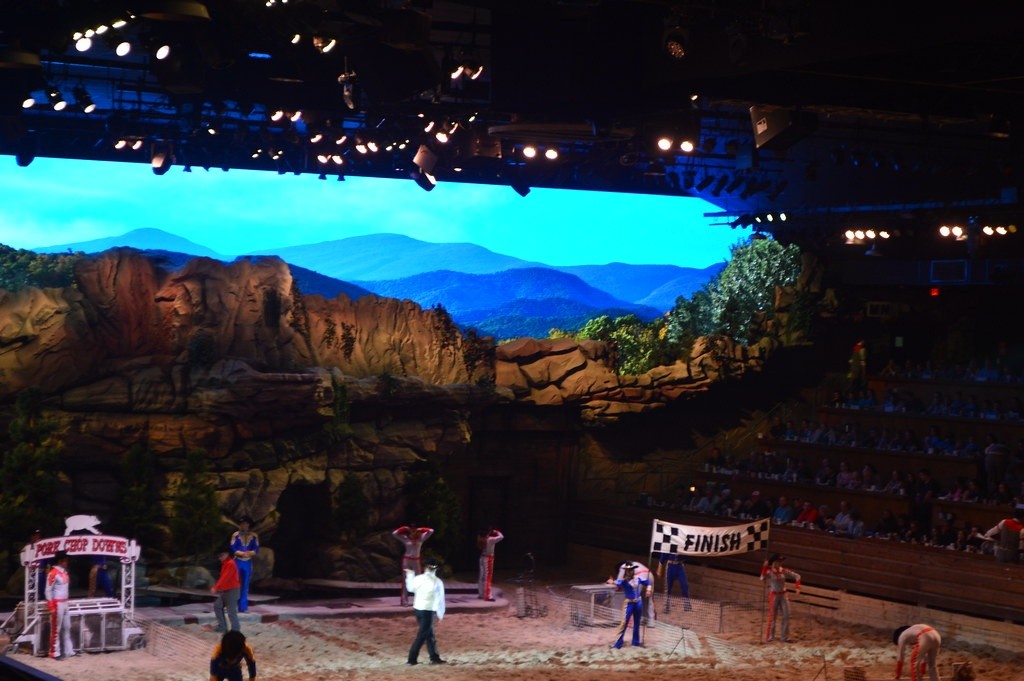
[422,558,442,570]
[622,562,638,570]
[768,554,786,565]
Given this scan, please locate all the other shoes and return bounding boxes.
[478,596,484,598]
[430,658,447,664]
[49,653,63,659]
[212,625,226,632]
[65,652,79,656]
[484,598,496,601]
[408,660,418,665]
[401,603,408,607]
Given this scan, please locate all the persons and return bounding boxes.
[608,558,657,626]
[210,542,241,634]
[648,334,1024,562]
[391,520,434,607]
[88,556,114,598]
[44,551,81,662]
[475,520,505,602]
[608,562,652,651]
[209,628,257,681]
[230,516,259,613]
[759,552,801,643]
[657,550,695,615]
[27,528,52,602]
[892,623,941,681]
[402,559,448,665]
